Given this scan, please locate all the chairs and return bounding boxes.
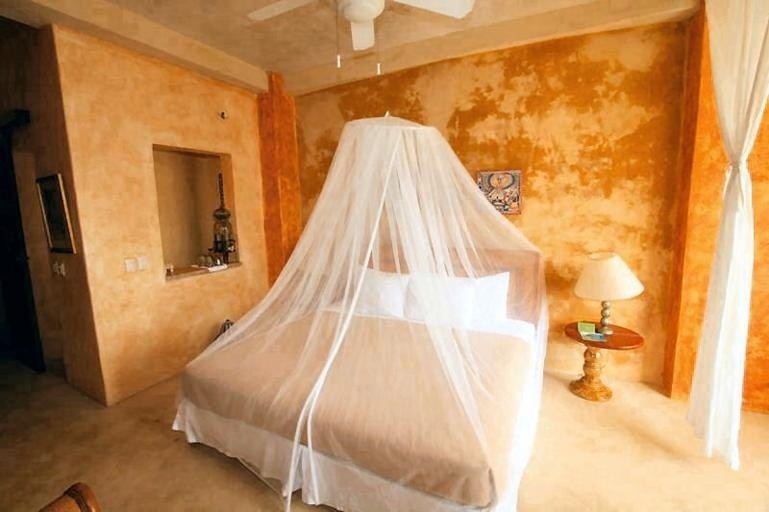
[39,482,102,512]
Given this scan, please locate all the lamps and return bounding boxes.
[335,1,384,75]
[573,252,645,334]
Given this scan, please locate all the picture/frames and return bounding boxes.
[35,172,76,254]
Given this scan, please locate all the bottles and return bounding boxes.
[197,247,222,267]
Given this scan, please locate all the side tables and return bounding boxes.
[565,320,644,402]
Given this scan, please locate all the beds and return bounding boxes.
[171,241,545,511]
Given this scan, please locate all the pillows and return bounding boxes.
[348,262,411,320]
[407,267,510,336]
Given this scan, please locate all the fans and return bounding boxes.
[247,0,479,51]
